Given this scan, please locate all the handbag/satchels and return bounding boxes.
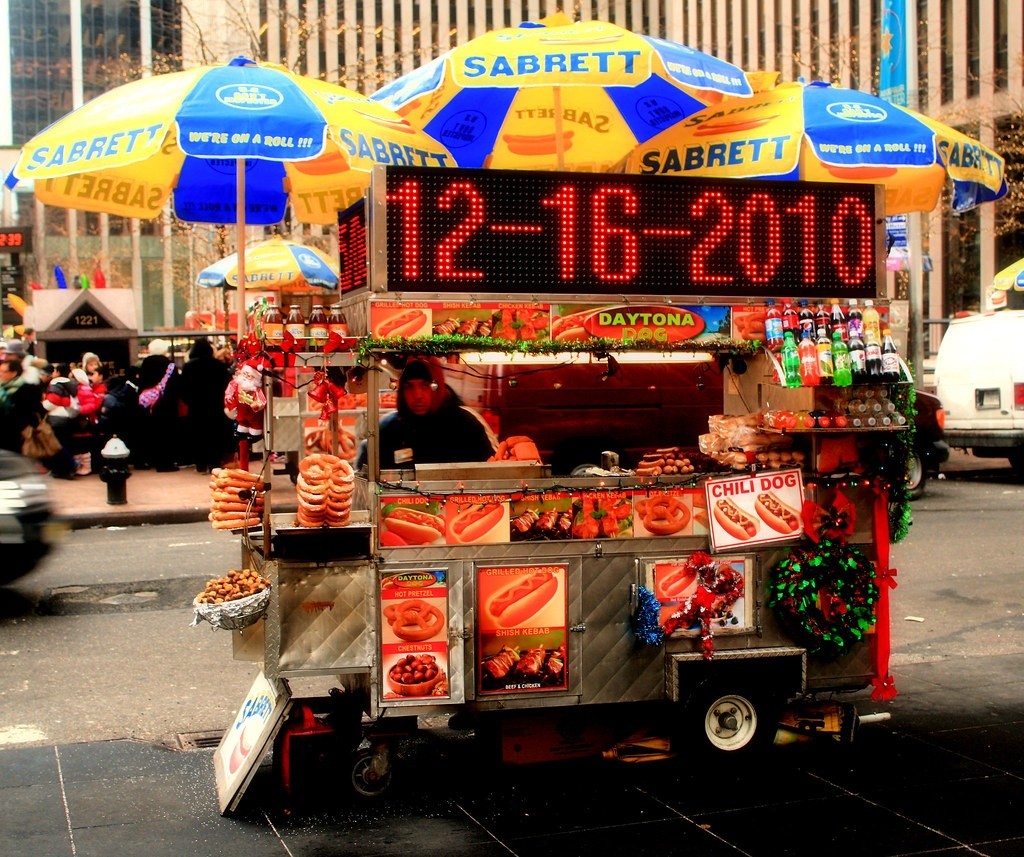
[139,363,176,414]
[22,413,62,458]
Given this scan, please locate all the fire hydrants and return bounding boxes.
[99,433,134,505]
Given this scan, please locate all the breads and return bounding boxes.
[208,467,263,529]
[635,495,692,535]
[698,413,809,469]
[383,599,444,642]
[296,453,356,528]
[734,312,768,342]
[305,428,359,461]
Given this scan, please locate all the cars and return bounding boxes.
[436,348,950,501]
[0,448,62,588]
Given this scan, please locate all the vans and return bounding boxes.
[934,308,1024,474]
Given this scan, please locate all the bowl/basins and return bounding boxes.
[387,663,443,697]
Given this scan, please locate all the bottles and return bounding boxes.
[760,298,906,430]
[246,297,349,352]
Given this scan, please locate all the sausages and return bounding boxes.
[637,447,695,477]
[495,436,533,461]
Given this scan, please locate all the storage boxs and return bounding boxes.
[724,354,851,419]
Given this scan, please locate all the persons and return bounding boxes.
[353,355,500,481]
[0,327,234,475]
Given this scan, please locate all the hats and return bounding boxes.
[71,368,90,386]
[148,339,172,355]
[82,352,102,372]
[402,361,432,383]
[6,339,23,354]
[57,365,70,377]
[40,365,54,377]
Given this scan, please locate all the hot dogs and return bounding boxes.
[376,310,427,340]
[551,307,605,343]
[660,567,697,597]
[487,570,558,628]
[713,490,803,540]
[384,502,504,543]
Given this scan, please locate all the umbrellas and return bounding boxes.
[605,78,1009,216]
[994,257,1024,292]
[3,53,458,343]
[198,235,339,306]
[366,11,779,174]
